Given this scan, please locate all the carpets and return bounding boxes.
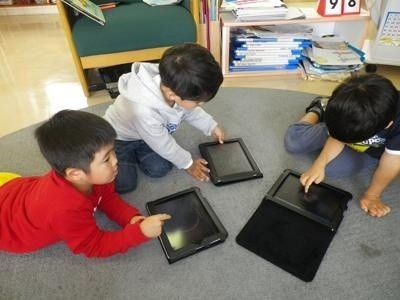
[0,88,400,300]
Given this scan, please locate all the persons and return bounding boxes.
[101,40,226,196]
[0,109,174,261]
[284,75,400,218]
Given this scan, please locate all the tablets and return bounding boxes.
[199,137,261,184]
[146,186,225,260]
[269,169,352,229]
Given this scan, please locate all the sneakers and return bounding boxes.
[305,96,331,111]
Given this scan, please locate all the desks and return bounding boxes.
[219,12,371,79]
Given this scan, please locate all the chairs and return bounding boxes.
[57,1,200,97]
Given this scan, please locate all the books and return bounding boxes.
[0,0,121,27]
[182,0,366,83]
[98,58,163,100]
